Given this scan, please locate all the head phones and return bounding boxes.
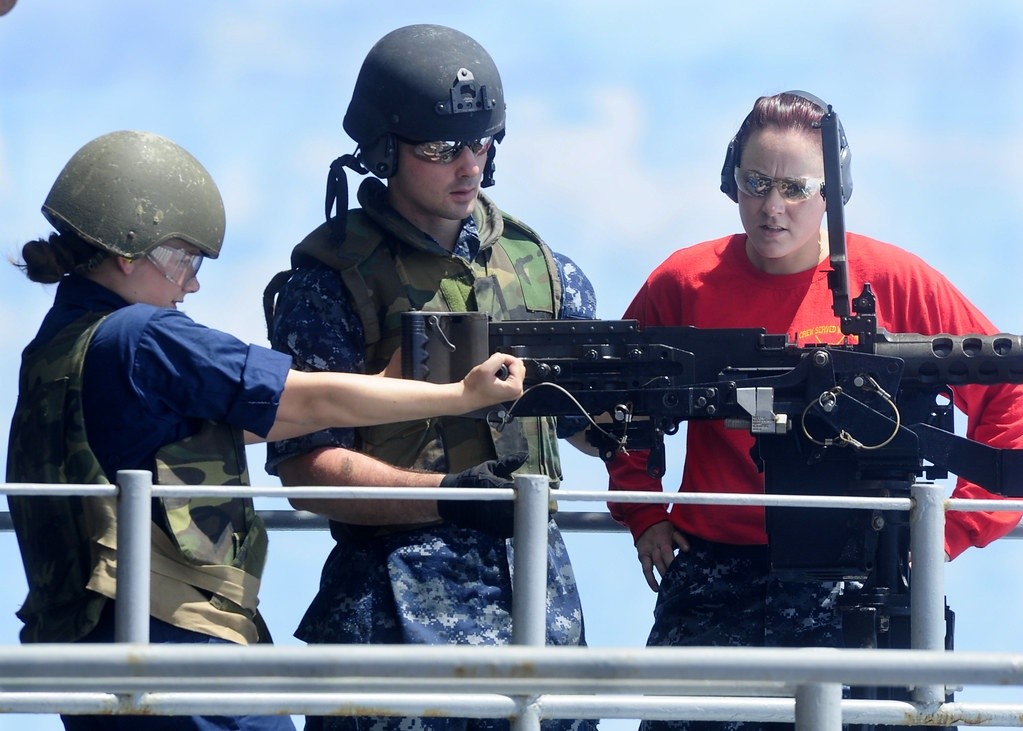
[720,90,853,207]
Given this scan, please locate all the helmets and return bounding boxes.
[343,24,506,150]
[40,130,226,259]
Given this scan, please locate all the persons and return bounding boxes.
[606,93,1022,731]
[265,24,618,731]
[5,131,527,731]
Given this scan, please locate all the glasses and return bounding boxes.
[413,136,494,164]
[734,164,825,204]
[145,244,203,289]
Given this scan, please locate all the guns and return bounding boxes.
[403,106,1022,500]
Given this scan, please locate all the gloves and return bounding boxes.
[437,451,530,538]
